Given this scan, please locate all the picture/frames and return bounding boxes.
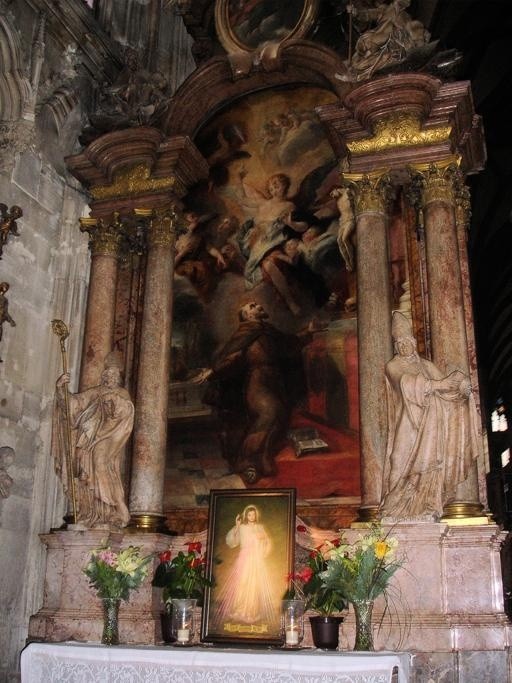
[200,488,298,646]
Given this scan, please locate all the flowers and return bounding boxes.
[287,538,349,617]
[152,541,216,599]
[322,525,419,651]
[82,543,154,602]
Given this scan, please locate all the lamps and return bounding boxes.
[171,599,197,646]
[280,599,304,649]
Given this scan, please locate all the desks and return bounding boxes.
[20,642,411,683]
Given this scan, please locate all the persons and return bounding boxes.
[95,45,168,128]
[386,334,481,518]
[49,366,136,532]
[207,504,278,632]
[0,201,25,260]
[1,280,17,363]
[346,0,433,67]
[0,445,16,500]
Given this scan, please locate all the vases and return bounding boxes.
[352,599,375,651]
[100,600,119,644]
[160,610,193,644]
[306,618,345,650]
